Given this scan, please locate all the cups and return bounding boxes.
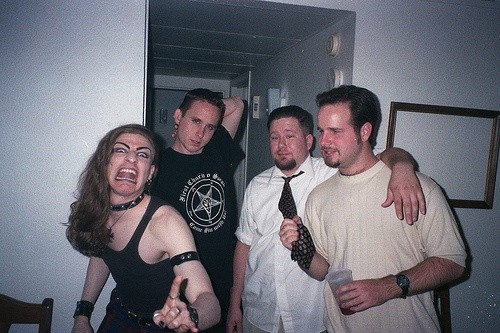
[325,269,356,315]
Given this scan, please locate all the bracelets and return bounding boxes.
[74,300,94,318]
[187,308,198,328]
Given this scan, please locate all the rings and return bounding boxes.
[175,307,181,313]
[153,312,160,318]
[169,293,180,299]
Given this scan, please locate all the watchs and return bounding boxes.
[397,274,410,297]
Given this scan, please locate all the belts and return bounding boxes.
[114,284,163,321]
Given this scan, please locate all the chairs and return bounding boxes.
[0,293,56,333]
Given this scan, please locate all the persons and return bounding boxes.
[279,85,467,333]
[150,88,242,333]
[226,106,426,333]
[66,124,221,333]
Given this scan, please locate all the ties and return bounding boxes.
[278,171,316,270]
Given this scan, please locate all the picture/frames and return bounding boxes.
[384,99,500,212]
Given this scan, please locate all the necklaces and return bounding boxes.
[109,194,144,212]
[106,198,137,233]
